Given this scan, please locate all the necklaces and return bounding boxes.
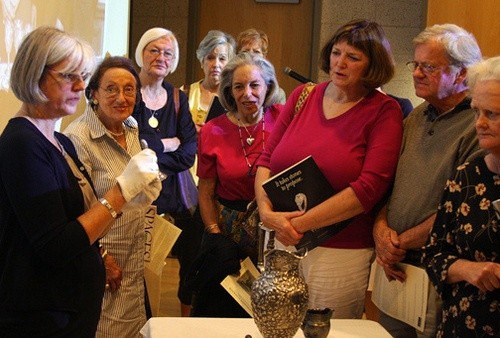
[237,112,258,146]
[143,84,161,128]
[239,107,265,177]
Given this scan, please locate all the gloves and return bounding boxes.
[122,180,162,210]
[115,148,159,203]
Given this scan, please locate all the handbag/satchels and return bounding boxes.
[183,233,238,291]
[177,168,199,210]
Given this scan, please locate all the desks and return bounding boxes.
[140,317,394,338]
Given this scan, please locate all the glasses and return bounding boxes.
[98,85,138,96]
[405,61,452,74]
[47,71,91,83]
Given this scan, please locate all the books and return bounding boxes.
[261,156,355,250]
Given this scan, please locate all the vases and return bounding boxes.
[252,246,309,338]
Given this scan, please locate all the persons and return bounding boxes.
[196,52,284,318]
[180,31,235,189]
[421,56,500,338]
[365,24,480,338]
[133,28,198,215]
[0,24,159,338]
[254,18,404,320]
[236,28,286,107]
[62,56,146,338]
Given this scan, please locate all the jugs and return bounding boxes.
[251,247,310,338]
[302,307,334,338]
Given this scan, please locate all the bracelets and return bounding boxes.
[99,198,117,218]
[204,222,219,232]
